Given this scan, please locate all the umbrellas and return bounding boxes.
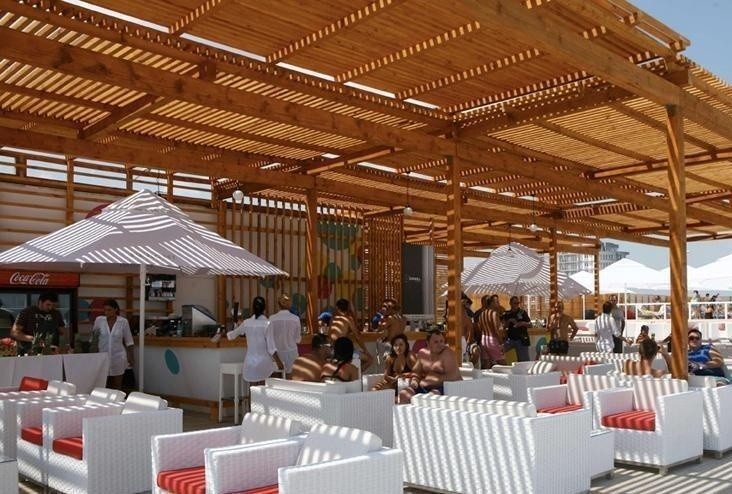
[626,266,711,319]
[1,189,289,392]
[696,254,732,292]
[566,270,600,319]
[583,256,659,321]
[440,241,592,319]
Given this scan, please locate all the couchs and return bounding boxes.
[444,374,560,401]
[363,372,494,401]
[391,392,614,493]
[579,352,639,359]
[250,376,395,448]
[510,355,613,383]
[660,374,732,459]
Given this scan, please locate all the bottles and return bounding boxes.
[75,333,80,352]
[169,320,191,338]
[59,332,66,354]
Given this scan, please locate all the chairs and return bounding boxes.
[152,413,301,493]
[0,375,48,394]
[16,387,126,484]
[45,391,183,494]
[0,379,76,458]
[594,379,705,475]
[527,374,625,415]
[212,422,404,494]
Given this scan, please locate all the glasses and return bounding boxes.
[690,336,699,340]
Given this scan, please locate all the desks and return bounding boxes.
[218,363,287,424]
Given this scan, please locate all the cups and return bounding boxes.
[156,329,160,338]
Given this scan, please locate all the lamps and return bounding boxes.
[404,171,414,218]
[530,194,538,234]
[233,181,242,204]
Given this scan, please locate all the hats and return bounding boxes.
[318,312,331,321]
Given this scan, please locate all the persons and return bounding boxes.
[594,294,632,354]
[218,295,284,414]
[624,339,672,379]
[685,329,726,378]
[636,325,655,344]
[86,299,134,397]
[269,295,303,380]
[288,290,533,406]
[651,291,725,319]
[9,288,65,355]
[543,300,579,356]
[660,331,673,353]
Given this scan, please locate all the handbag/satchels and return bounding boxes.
[520,335,530,346]
[123,368,137,387]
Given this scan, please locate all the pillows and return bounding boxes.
[527,360,556,375]
